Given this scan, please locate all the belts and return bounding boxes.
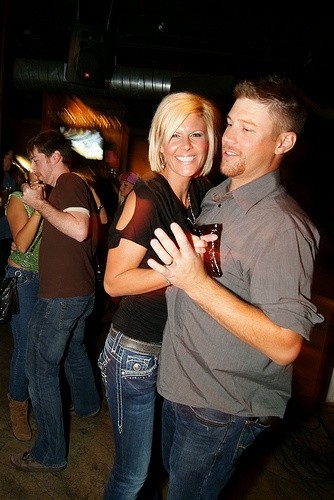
[109,324,162,354]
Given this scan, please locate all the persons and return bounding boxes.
[0,95,144,473]
[97,92,220,500]
[147,74,325,500]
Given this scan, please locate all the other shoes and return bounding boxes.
[11,450,66,473]
[64,401,100,420]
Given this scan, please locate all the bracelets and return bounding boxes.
[97,200,104,210]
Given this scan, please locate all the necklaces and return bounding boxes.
[183,193,197,232]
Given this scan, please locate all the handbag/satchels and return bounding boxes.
[0,274,20,326]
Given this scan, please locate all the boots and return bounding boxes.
[8,393,31,442]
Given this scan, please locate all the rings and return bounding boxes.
[165,259,173,266]
